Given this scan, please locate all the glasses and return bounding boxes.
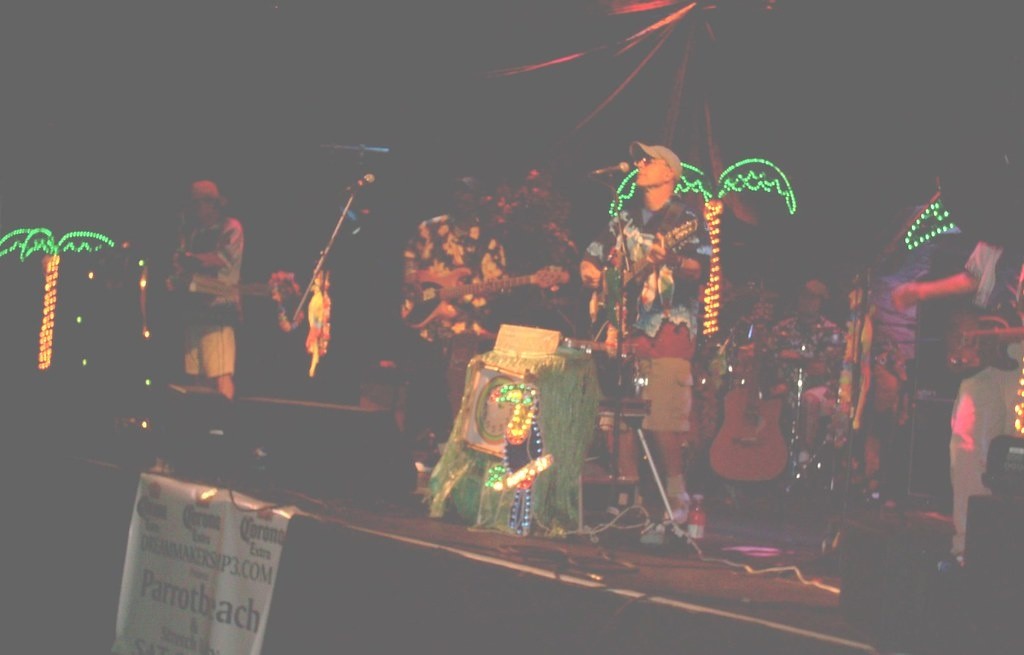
[634,157,661,166]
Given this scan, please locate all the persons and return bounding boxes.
[738,278,847,471]
[890,227,1024,573]
[399,175,507,480]
[172,180,244,400]
[579,141,711,529]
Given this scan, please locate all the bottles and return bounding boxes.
[687,495,706,542]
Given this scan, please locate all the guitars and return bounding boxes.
[589,220,698,356]
[402,265,570,330]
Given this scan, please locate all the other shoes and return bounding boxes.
[663,492,690,524]
[607,494,642,515]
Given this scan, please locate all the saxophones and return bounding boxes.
[789,343,845,481]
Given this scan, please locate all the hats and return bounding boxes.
[630,141,682,176]
[188,181,228,207]
[801,281,829,299]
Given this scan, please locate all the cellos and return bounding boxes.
[710,273,788,481]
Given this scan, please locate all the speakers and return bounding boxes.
[233,398,418,497]
[162,384,233,465]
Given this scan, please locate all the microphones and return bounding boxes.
[346,174,375,190]
[593,162,629,174]
